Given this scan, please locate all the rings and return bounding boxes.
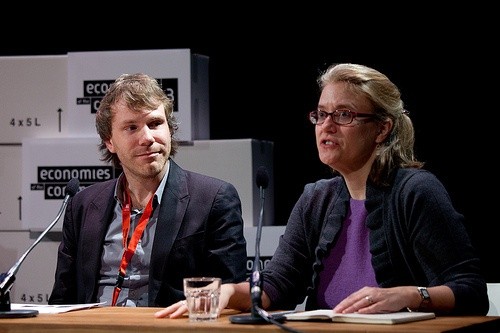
[366,296,374,305]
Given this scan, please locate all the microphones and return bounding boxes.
[1,178,81,318]
[229,167,286,324]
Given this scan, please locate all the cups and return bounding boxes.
[183,277,222,322]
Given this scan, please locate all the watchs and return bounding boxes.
[417,286,431,312]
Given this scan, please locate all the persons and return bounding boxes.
[46,72,249,308]
[155,62,491,320]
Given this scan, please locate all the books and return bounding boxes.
[282,309,436,325]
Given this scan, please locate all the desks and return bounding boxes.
[0,303,500,333]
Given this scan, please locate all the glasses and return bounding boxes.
[309,110,380,125]
